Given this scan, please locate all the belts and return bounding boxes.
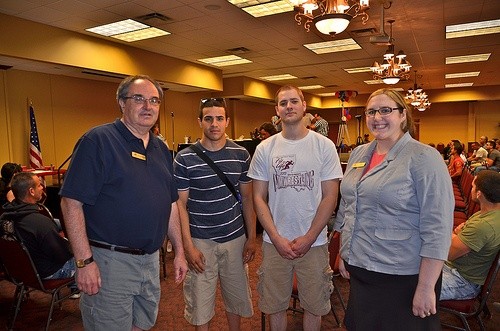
[88,240,147,255]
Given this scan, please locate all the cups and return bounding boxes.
[183,135,188,144]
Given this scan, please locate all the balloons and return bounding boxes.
[341,108,351,122]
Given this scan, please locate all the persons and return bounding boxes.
[440,170,500,301]
[444,135,500,179]
[0,172,81,299]
[0,162,22,211]
[170,97,257,331]
[246,86,343,331]
[260,111,329,141]
[338,89,455,331]
[59,75,188,331]
[341,133,370,153]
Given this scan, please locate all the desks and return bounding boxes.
[23,168,67,188]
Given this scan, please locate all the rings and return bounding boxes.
[426,313,430,315]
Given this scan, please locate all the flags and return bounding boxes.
[29,105,47,205]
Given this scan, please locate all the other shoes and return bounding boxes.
[70,288,81,298]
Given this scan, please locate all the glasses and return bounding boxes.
[200,97,226,106]
[122,95,161,106]
[365,107,403,116]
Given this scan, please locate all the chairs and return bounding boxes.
[0,220,82,331]
[436,142,481,232]
[439,250,500,331]
[261,231,346,331]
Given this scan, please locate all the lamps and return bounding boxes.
[293,0,371,39]
[403,70,432,112]
[369,19,412,87]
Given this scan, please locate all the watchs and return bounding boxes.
[76,256,94,268]
